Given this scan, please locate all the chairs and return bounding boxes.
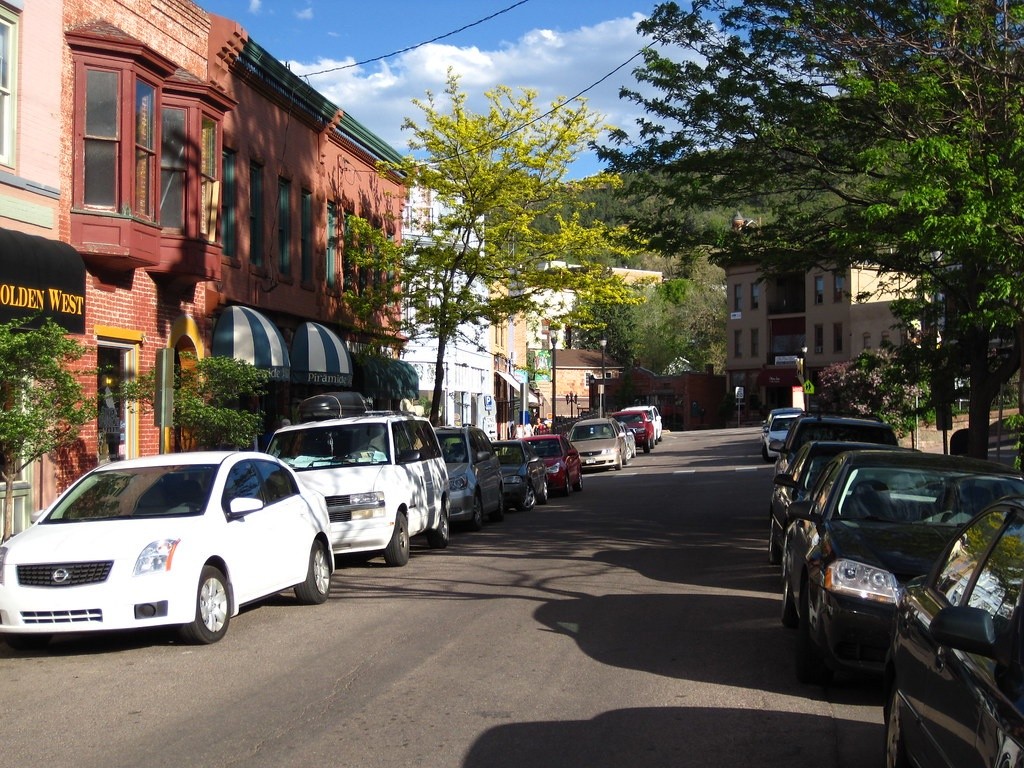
[842,480,895,520]
[172,479,201,506]
[449,443,464,457]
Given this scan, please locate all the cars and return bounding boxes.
[760,406,805,463]
[880,498,1024,768]
[489,440,550,512]
[611,410,654,454]
[616,421,636,461]
[519,434,583,498]
[1,451,336,651]
[768,441,925,565]
[781,450,1024,684]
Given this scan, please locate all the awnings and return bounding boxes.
[0,227,87,335]
[495,371,543,406]
[212,304,419,400]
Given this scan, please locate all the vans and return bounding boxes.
[264,409,451,567]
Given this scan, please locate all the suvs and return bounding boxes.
[429,422,505,532]
[621,405,662,445]
[567,416,626,471]
[769,412,900,490]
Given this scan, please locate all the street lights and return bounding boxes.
[548,324,561,425]
[599,332,606,418]
[566,392,578,418]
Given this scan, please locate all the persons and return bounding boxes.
[281,419,291,426]
[533,417,545,431]
